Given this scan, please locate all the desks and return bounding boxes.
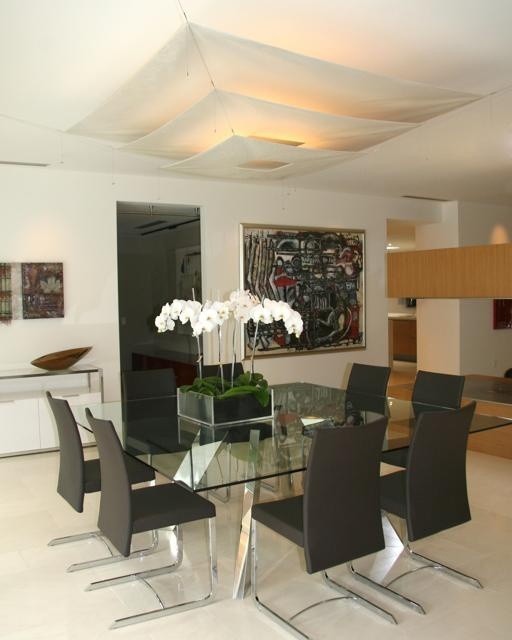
[0,363,103,461]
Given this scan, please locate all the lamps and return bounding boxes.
[64,1,489,185]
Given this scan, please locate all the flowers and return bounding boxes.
[153,289,304,399]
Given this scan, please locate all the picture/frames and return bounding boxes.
[239,220,369,361]
[20,262,65,319]
